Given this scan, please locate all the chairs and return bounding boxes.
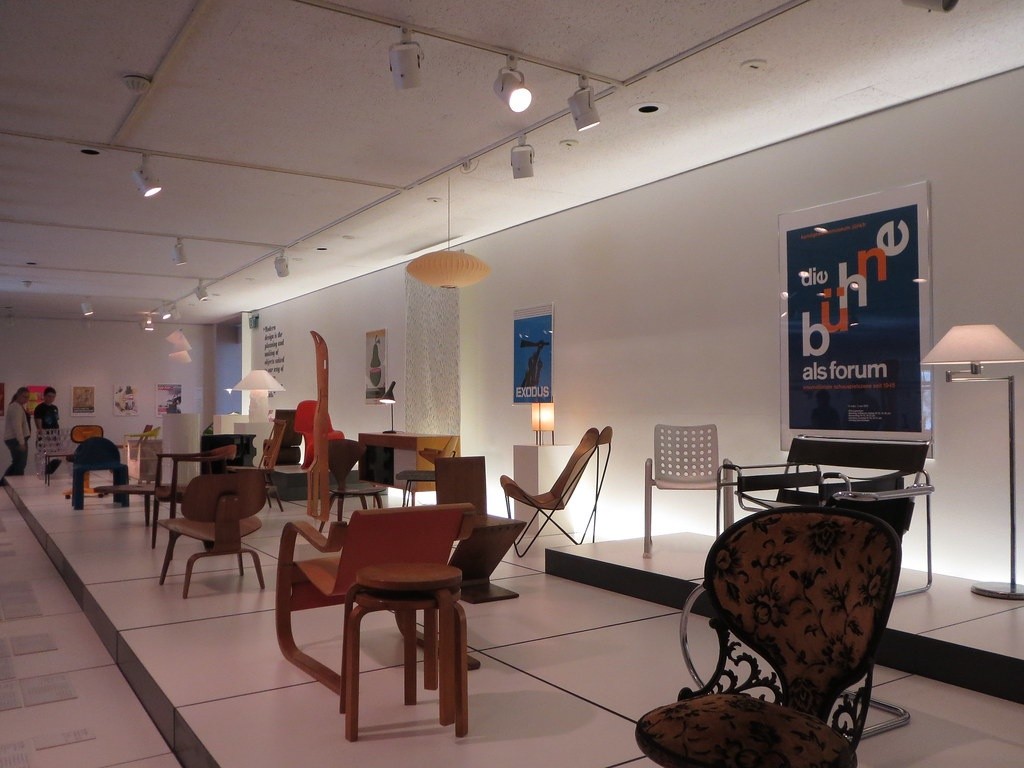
[156,469,267,598]
[318,437,385,533]
[43,424,161,510]
[679,484,936,741]
[395,436,459,507]
[500,425,613,557]
[643,422,734,557]
[275,503,480,695]
[717,435,936,596]
[151,444,237,549]
[435,454,528,604]
[634,505,901,768]
[228,420,288,510]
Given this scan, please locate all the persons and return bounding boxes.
[0,387,32,487]
[34,387,60,472]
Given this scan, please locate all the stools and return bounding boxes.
[340,562,469,741]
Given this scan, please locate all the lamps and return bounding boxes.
[387,21,603,289]
[80,155,288,332]
[231,371,286,423]
[531,396,555,446]
[379,381,397,433]
[920,324,1024,602]
